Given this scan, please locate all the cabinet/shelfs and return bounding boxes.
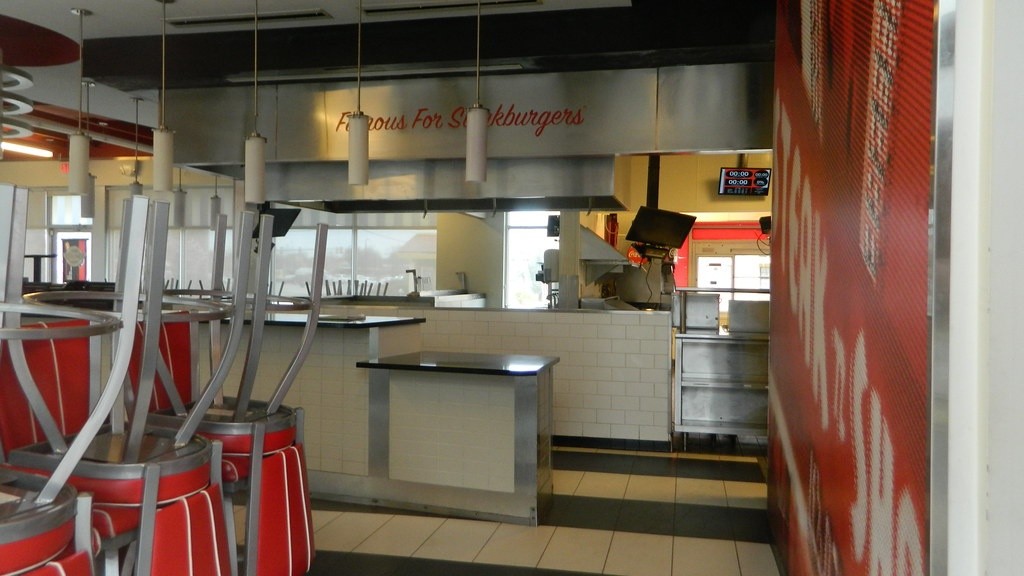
[671,285,771,451]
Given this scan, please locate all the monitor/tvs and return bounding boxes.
[718,167,771,196]
[252,209,301,238]
[625,205,697,249]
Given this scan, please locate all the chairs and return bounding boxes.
[0,181,331,576]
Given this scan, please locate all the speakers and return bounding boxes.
[759,216,771,234]
[547,215,560,237]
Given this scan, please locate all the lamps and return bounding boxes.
[66,8,93,196]
[243,0,268,204]
[464,0,487,182]
[347,0,370,185]
[127,98,145,195]
[79,81,95,218]
[151,0,177,192]
